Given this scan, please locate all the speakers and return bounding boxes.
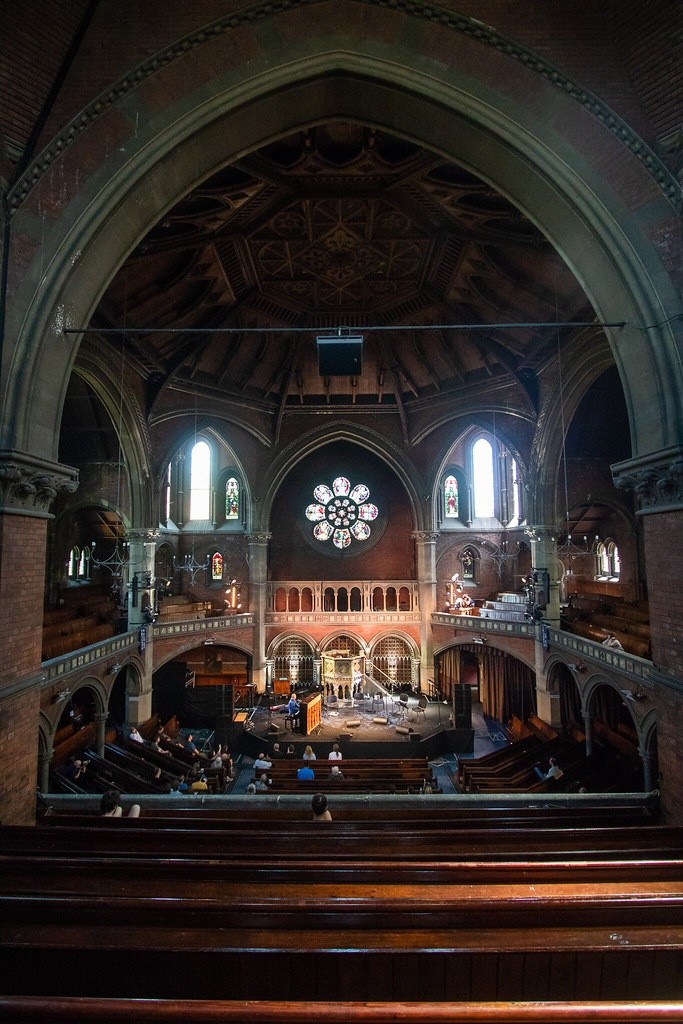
[373,717,388,725]
[270,723,279,732]
[410,734,420,741]
[396,726,413,735]
[268,733,279,741]
[340,733,350,741]
[347,720,361,728]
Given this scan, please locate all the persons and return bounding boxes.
[310,793,333,820]
[100,790,141,817]
[288,694,301,718]
[72,724,345,795]
[365,784,432,794]
[455,593,475,607]
[602,632,625,651]
[544,758,564,780]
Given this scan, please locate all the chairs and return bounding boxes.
[326,696,339,713]
[394,693,409,720]
[372,692,384,711]
[410,699,427,723]
[352,693,365,713]
[285,716,298,731]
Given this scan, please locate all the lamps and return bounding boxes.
[54,688,71,704]
[156,577,170,589]
[172,386,210,587]
[538,252,602,583]
[521,575,532,584]
[204,636,216,646]
[472,637,489,646]
[109,663,123,674]
[620,690,643,703]
[479,402,521,578]
[569,664,584,673]
[90,269,148,596]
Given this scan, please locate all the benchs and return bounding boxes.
[42,585,123,660]
[250,757,443,794]
[454,713,644,794]
[50,715,227,794]
[560,590,651,660]
[0,804,683,1024]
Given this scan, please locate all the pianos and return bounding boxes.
[293,690,322,736]
